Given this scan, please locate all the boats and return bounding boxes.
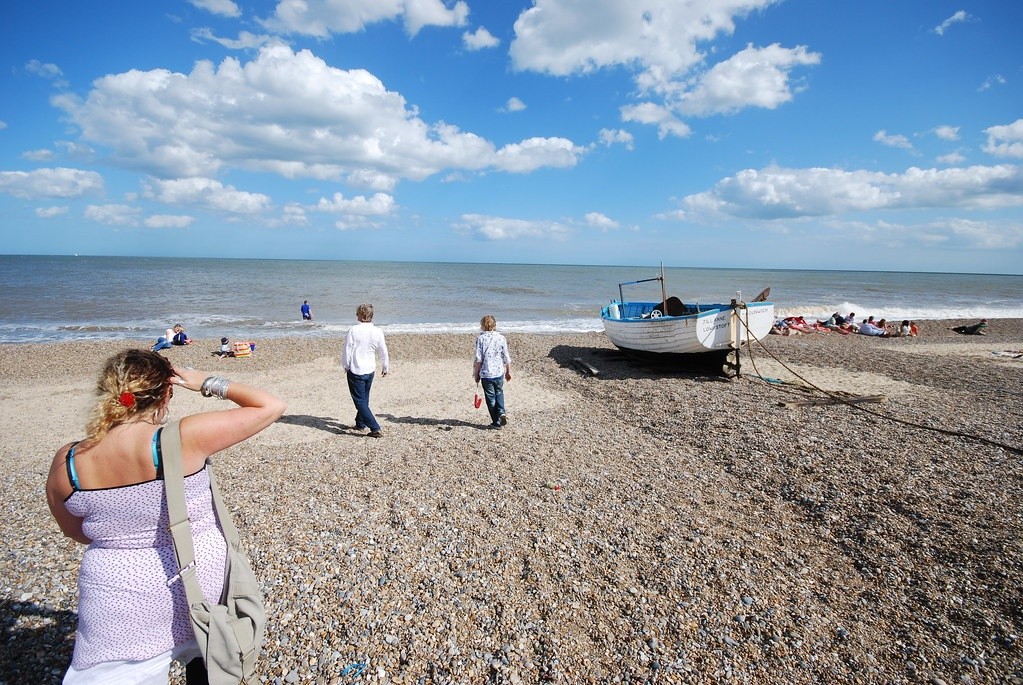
[599,261,775,357]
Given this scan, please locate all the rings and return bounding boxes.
[187,366,193,370]
[178,381,184,386]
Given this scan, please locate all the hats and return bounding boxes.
[221,337,229,345]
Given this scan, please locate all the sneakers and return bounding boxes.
[499,414,507,426]
[490,422,502,430]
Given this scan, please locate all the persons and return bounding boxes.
[45,347,287,685]
[772,316,812,335]
[147,324,193,352]
[948,319,989,336]
[474,316,511,429]
[217,338,235,361]
[300,300,314,321]
[897,320,918,338]
[817,313,856,334]
[342,304,390,437]
[859,316,891,337]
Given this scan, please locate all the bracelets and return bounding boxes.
[201,376,233,400]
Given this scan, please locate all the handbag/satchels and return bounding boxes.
[161,418,266,685]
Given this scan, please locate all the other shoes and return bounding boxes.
[367,432,384,437]
[354,425,364,431]
